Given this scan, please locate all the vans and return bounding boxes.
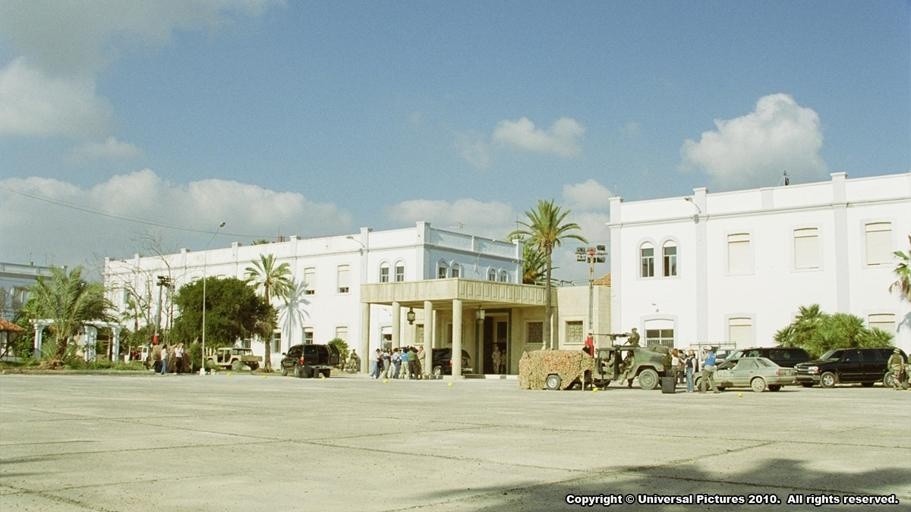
[718,347,811,370]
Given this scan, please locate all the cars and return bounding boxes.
[794,348,908,388]
[692,357,795,392]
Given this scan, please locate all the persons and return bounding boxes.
[130,333,199,375]
[888,348,905,383]
[618,350,635,373]
[491,346,501,374]
[671,344,720,394]
[586,333,594,356]
[624,327,640,346]
[370,346,426,381]
[234,337,242,348]
[500,349,507,374]
[337,349,357,372]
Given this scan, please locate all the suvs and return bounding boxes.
[593,346,671,389]
[281,345,338,378]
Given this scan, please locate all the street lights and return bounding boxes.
[200,223,225,377]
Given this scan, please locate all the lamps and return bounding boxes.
[476,305,486,325]
[407,306,416,325]
